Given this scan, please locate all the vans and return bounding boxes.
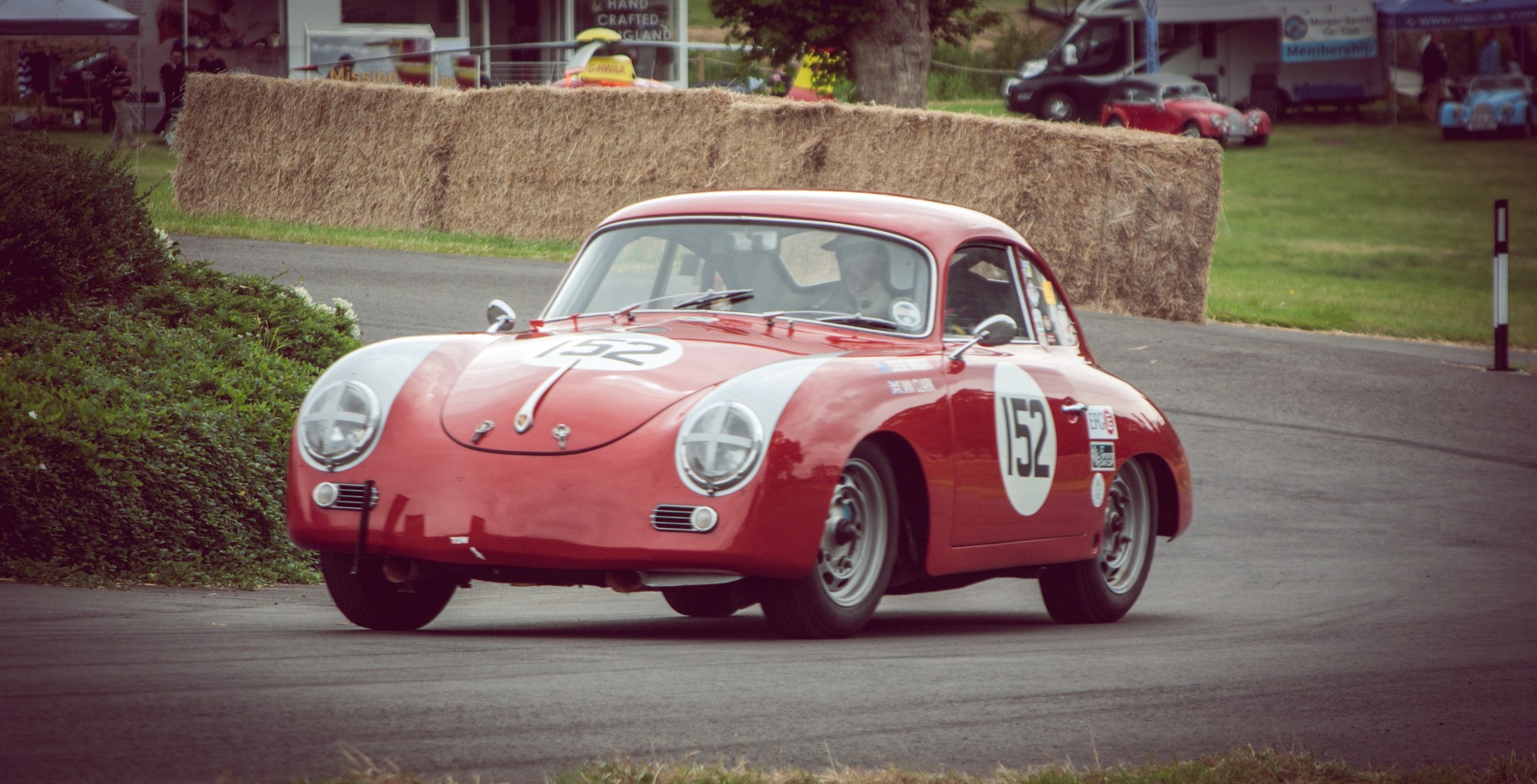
[1004,0,1383,124]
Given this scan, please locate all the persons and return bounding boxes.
[196,42,229,74]
[152,46,189,138]
[813,243,894,322]
[97,46,120,133]
[1419,24,1520,121]
[766,62,793,97]
[327,53,355,79]
[102,55,146,154]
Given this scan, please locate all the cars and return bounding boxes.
[1100,72,1274,150]
[1440,73,1536,141]
[283,185,1193,640]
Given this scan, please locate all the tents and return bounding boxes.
[0,0,141,135]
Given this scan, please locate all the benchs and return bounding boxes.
[1292,83,1379,122]
